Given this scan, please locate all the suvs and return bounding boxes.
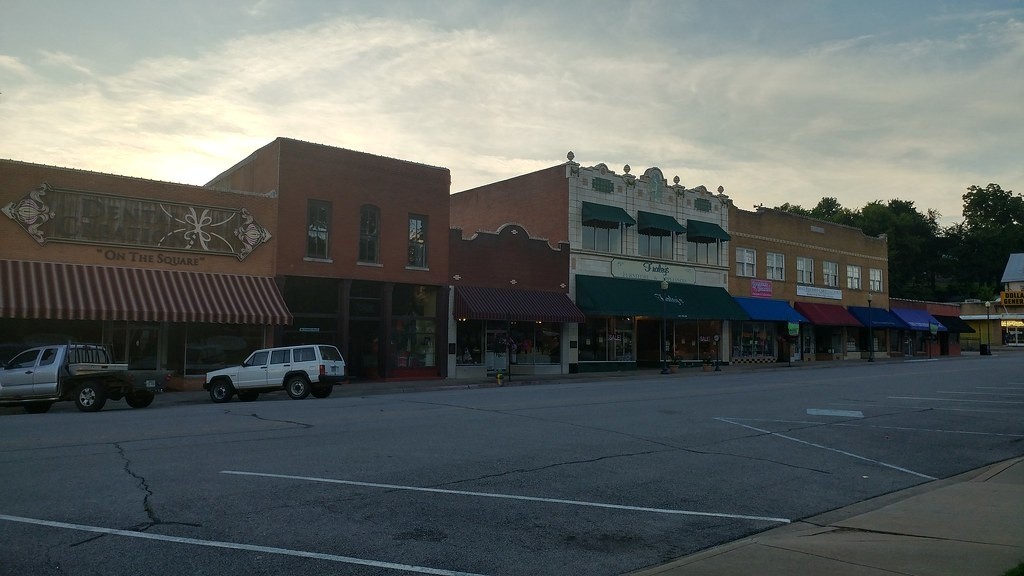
[203,343,347,404]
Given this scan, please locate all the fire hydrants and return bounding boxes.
[496,368,507,387]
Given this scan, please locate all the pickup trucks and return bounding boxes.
[0,341,175,412]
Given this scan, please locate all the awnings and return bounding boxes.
[732,296,811,323]
[575,273,752,321]
[686,219,732,241]
[0,259,294,328]
[582,200,636,225]
[453,284,587,324]
[794,301,865,328]
[890,307,948,332]
[847,305,911,330]
[931,314,976,333]
[638,210,687,234]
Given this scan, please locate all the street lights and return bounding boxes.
[661,276,670,375]
[985,301,992,355]
[867,292,875,362]
